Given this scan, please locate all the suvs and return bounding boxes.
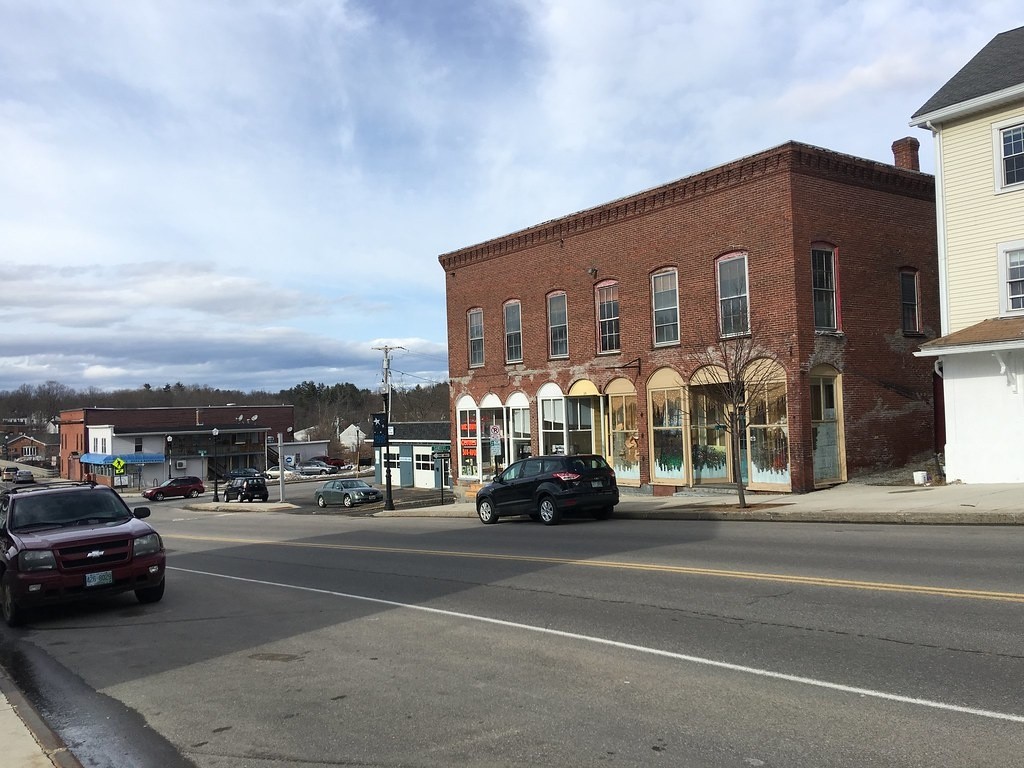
[0,479,167,628]
[476,453,619,526]
[296,461,338,476]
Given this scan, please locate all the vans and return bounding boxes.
[224,476,269,503]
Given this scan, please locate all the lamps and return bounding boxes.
[167,436,173,442]
[212,428,218,435]
[379,380,389,394]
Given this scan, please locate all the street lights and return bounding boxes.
[5,435,9,462]
[379,381,396,510]
[356,426,361,472]
[212,428,220,502]
[166,435,173,480]
[30,437,34,466]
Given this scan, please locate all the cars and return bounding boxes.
[313,479,383,508]
[1,466,20,483]
[227,468,261,479]
[142,475,204,502]
[340,462,354,470]
[263,465,300,479]
[12,471,34,484]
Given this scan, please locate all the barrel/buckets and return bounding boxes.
[913,470,928,484]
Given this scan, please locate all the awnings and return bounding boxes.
[79,453,165,465]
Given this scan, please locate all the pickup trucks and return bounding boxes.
[309,456,345,471]
